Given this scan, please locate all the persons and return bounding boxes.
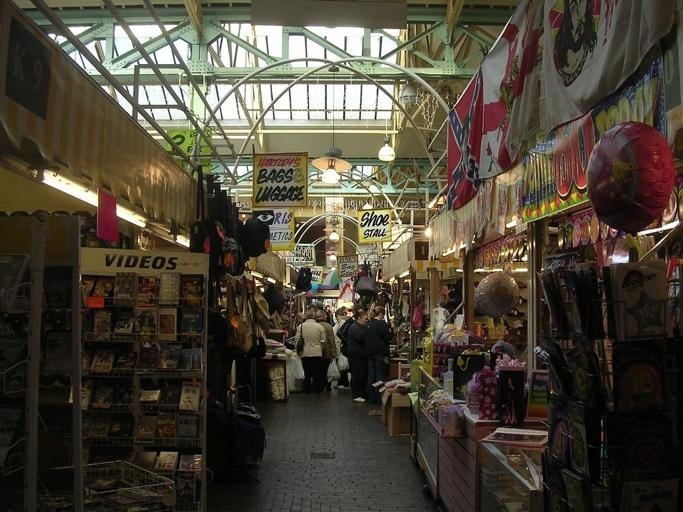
[283,302,391,405]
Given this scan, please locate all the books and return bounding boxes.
[82,274,205,509]
[479,425,551,447]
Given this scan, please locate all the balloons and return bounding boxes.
[474,271,520,321]
[584,121,678,236]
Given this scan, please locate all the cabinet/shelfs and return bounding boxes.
[0,244,311,510]
[374,231,681,510]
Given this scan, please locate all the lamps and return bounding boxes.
[308,63,351,273]
[361,74,423,215]
[27,165,191,252]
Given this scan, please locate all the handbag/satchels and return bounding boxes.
[337,318,355,343]
[451,350,496,420]
[190,218,270,276]
[297,335,304,356]
[352,271,374,295]
[215,313,267,361]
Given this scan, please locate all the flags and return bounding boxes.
[535,0,683,137]
[164,128,213,181]
[443,64,484,210]
[95,188,122,243]
[250,209,296,251]
[476,2,547,184]
[249,152,309,211]
[356,209,393,244]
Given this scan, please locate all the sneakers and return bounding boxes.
[352,397,366,402]
